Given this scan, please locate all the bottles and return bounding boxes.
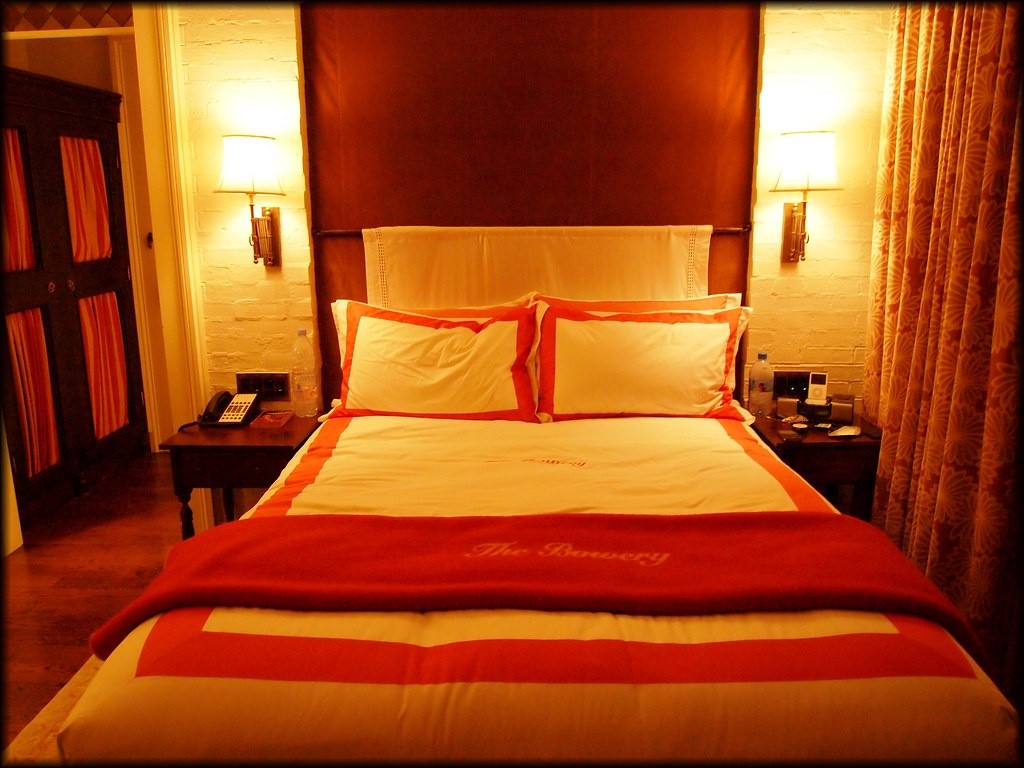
[749,352,774,416]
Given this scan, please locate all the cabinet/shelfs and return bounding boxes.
[1,65,152,514]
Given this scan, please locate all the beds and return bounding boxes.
[53,226,1020,765]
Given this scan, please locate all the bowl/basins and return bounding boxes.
[792,423,807,433]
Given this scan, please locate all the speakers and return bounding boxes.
[830,400,853,425]
[776,398,801,420]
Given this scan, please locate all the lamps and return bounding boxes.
[211,133,287,266]
[767,129,844,264]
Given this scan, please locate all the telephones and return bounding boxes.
[197,392,260,428]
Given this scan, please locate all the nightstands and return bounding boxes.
[159,413,322,542]
[751,414,883,516]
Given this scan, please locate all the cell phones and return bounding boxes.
[776,429,803,441]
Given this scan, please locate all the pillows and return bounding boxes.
[534,293,743,312]
[318,299,555,426]
[534,304,756,425]
[329,292,539,372]
